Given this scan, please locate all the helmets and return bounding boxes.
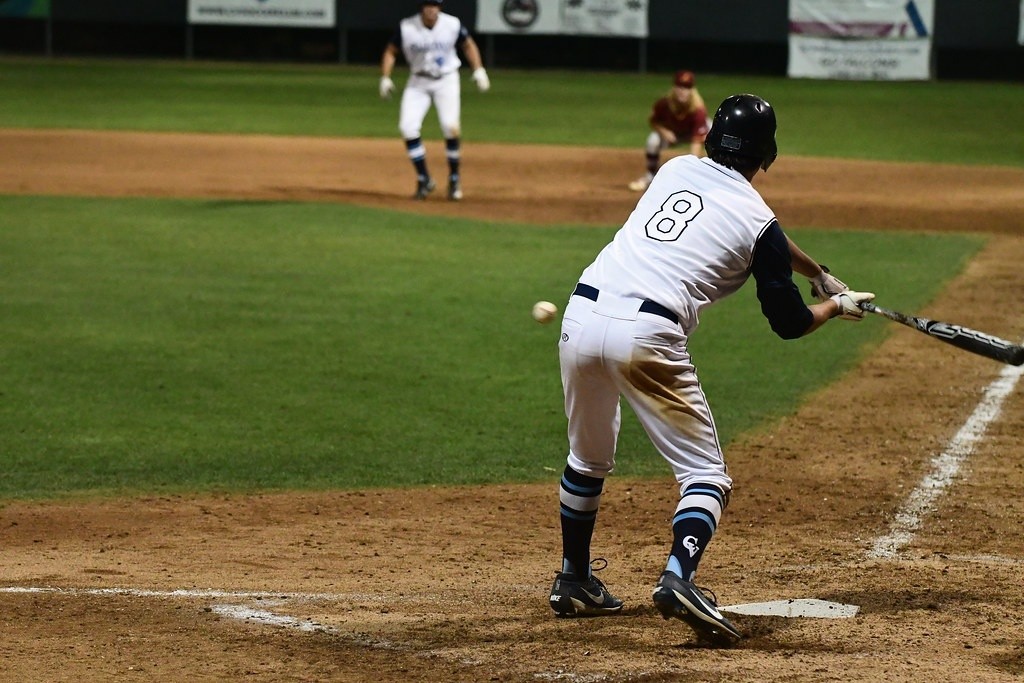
[706,94,779,172]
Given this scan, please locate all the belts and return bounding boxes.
[572,283,678,325]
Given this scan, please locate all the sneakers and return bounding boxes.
[653,571,741,648]
[550,557,623,617]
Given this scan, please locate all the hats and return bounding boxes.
[674,71,695,88]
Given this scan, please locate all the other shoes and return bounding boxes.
[449,182,462,200]
[630,177,651,192]
[416,183,434,198]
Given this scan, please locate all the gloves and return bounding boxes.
[470,68,491,91]
[807,266,849,301]
[830,285,877,321]
[379,76,396,101]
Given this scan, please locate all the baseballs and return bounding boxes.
[534,299,558,323]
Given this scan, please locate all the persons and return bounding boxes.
[549,94,875,640]
[629,70,713,190]
[379,0,490,201]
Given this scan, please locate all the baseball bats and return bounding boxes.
[810,285,1024,367]
